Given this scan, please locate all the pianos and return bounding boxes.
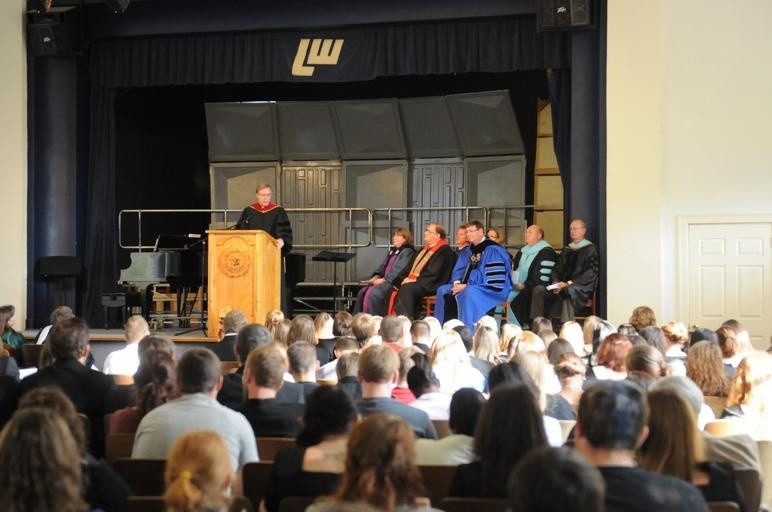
[117,245,201,327]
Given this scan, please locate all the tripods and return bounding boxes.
[175,219,239,337]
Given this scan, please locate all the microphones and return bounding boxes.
[239,215,253,226]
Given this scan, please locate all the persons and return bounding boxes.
[380,223,456,320]
[528,218,600,333]
[485,228,503,245]
[434,222,512,336]
[2,307,772,511]
[493,225,558,336]
[235,183,292,319]
[353,228,418,316]
[455,225,470,256]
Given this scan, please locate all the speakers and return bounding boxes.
[537,0,598,35]
[26,6,81,59]
